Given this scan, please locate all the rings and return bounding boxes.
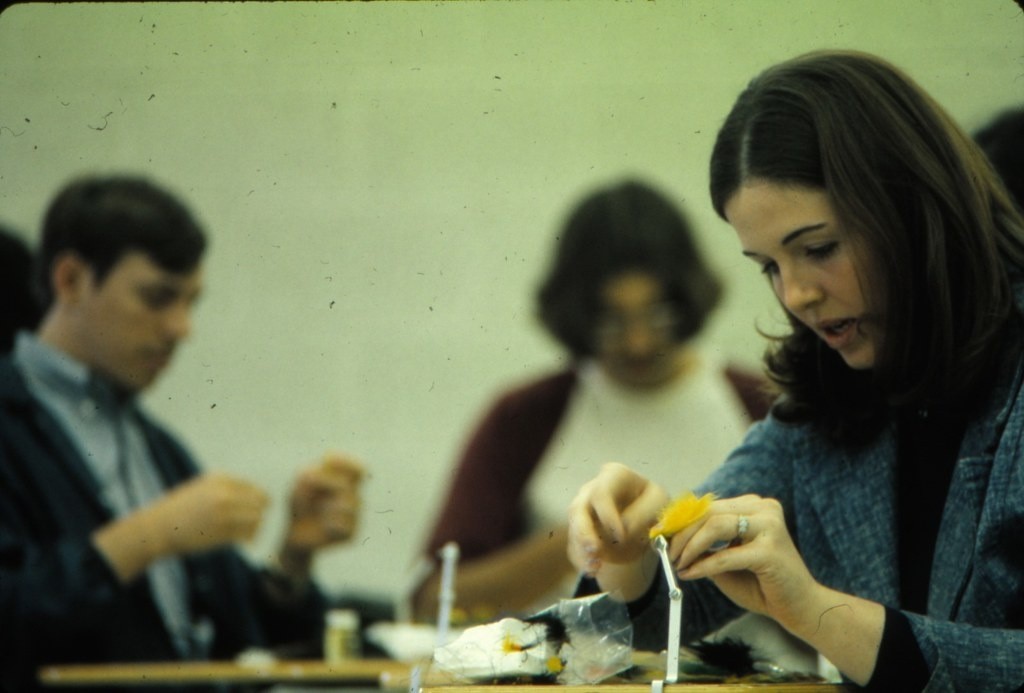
[738,514,750,538]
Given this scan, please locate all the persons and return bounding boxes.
[0,175,362,693]
[564,46,1024,693]
[404,180,782,628]
